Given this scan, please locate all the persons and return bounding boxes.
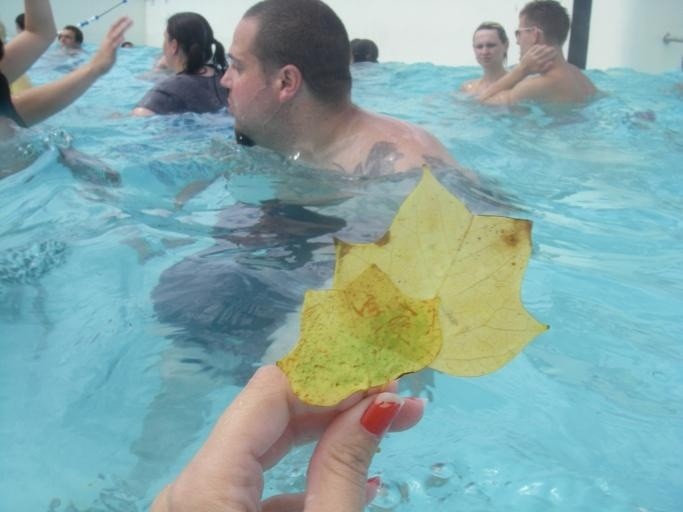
[49,0,492,512]
[460,19,511,97]
[480,0,595,112]
[149,363,427,512]
